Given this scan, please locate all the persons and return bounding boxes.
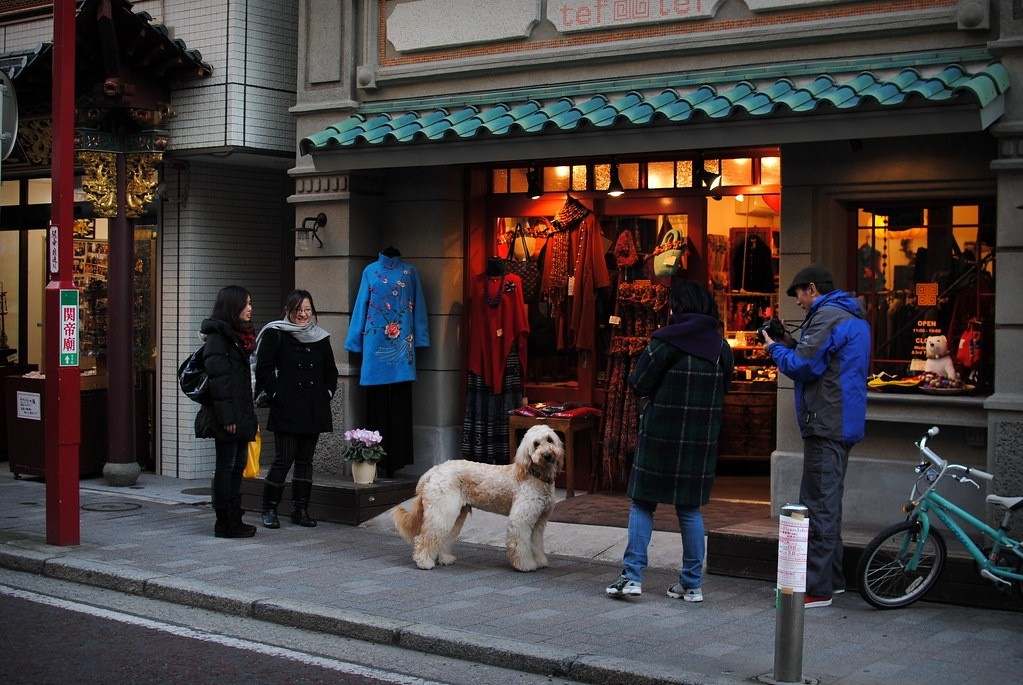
[194,286,259,539]
[760,263,872,609]
[605,276,735,603]
[257,290,338,529]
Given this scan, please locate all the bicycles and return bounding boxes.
[855,426,1023,610]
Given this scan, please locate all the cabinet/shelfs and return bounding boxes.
[716,293,778,468]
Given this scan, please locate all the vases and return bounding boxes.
[351,460,376,484]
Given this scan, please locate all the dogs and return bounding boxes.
[391,424,563,572]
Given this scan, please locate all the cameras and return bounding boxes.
[756,317,785,344]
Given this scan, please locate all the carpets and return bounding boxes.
[548,492,771,536]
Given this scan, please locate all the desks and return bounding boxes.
[509,415,599,500]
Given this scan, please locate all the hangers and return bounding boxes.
[861,236,870,249]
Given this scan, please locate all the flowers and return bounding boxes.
[341,428,387,466]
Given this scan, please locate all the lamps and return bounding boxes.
[606,163,625,197]
[695,167,722,191]
[526,171,543,200]
[867,215,929,240]
[292,212,327,248]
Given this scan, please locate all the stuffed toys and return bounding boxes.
[924,335,956,381]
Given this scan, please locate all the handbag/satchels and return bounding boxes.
[255,331,281,408]
[176,343,228,404]
[242,427,261,478]
[653,229,683,277]
[489,223,540,303]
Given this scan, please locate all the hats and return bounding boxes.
[786,264,831,297]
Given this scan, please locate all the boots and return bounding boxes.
[261,477,285,528]
[291,477,317,527]
[212,506,257,538]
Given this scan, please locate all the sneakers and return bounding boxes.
[804,593,833,608]
[667,581,703,602]
[833,589,845,594]
[606,568,641,596]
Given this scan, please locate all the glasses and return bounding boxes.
[297,308,312,314]
